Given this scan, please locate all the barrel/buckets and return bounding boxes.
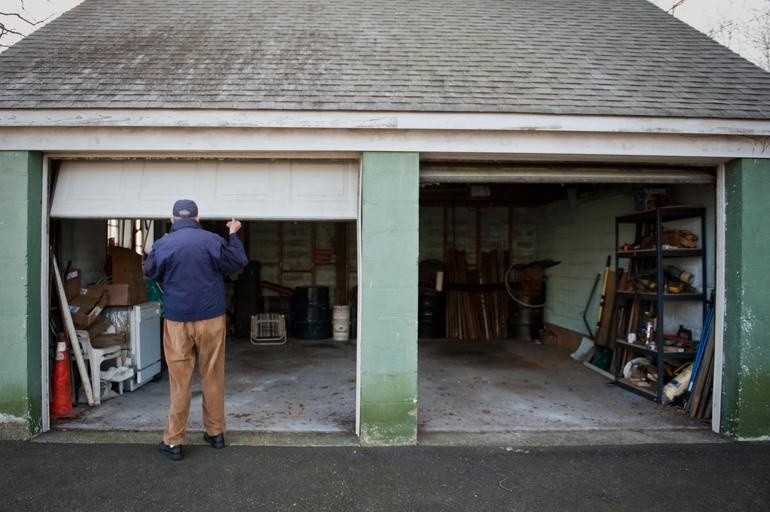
[333,304,350,341]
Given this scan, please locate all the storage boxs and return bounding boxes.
[63,246,146,347]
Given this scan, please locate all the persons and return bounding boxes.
[142,199,251,461]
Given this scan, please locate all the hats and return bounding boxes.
[171,199,199,219]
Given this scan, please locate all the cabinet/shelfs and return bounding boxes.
[103,301,162,391]
[616,207,706,405]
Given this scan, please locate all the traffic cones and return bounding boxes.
[49,334,82,419]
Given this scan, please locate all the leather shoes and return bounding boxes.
[203,430,225,450]
[158,440,182,460]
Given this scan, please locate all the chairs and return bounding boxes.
[70,330,124,406]
